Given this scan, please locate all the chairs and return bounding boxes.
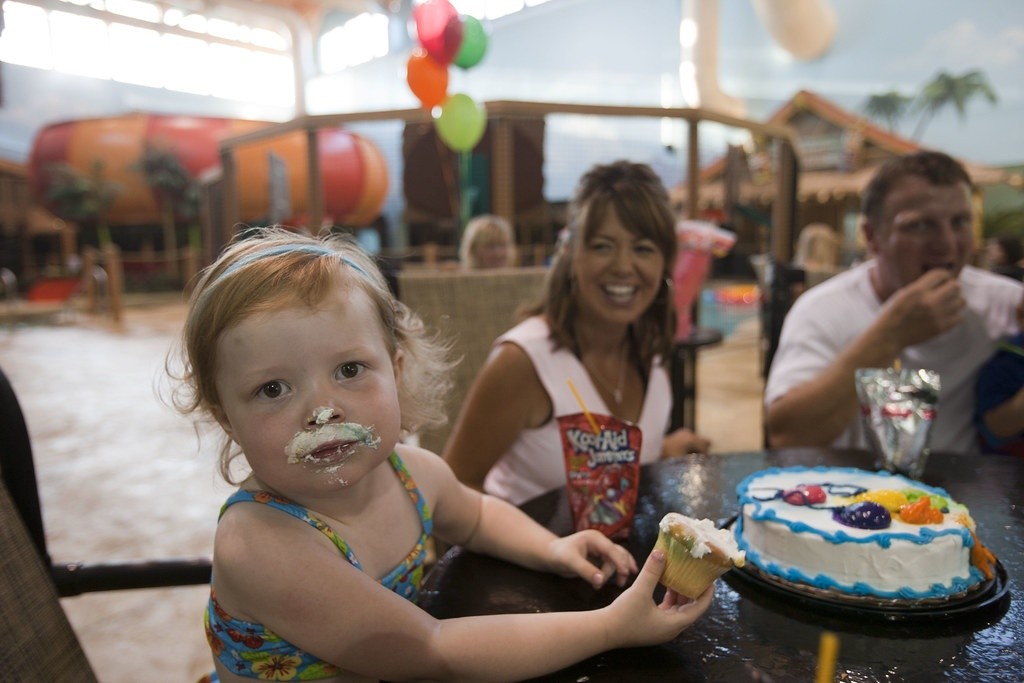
[396,269,548,456]
[748,249,807,449]
[0,365,211,683]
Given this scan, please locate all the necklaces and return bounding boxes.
[582,345,631,405]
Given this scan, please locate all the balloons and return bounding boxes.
[407,0,487,155]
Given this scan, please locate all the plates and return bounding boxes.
[720,512,1010,624]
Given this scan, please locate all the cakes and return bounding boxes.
[734,466,996,601]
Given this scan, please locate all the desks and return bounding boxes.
[358,446,1024,683]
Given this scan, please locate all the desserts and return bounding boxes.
[651,511,747,599]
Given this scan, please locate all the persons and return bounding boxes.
[441,161,711,504]
[459,152,1024,452]
[181,227,717,683]
